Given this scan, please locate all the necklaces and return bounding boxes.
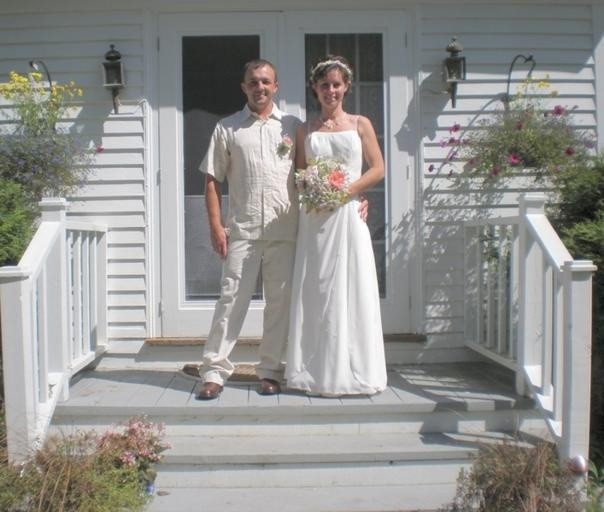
[318,110,346,130]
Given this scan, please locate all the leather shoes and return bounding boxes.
[199,382,223,399]
[256,378,281,395]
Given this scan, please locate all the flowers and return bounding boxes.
[281,133,293,151]
[295,156,351,213]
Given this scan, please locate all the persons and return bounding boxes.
[282,55,387,397]
[193,60,368,400]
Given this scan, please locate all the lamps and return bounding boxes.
[101,44,124,113]
[442,36,466,108]
